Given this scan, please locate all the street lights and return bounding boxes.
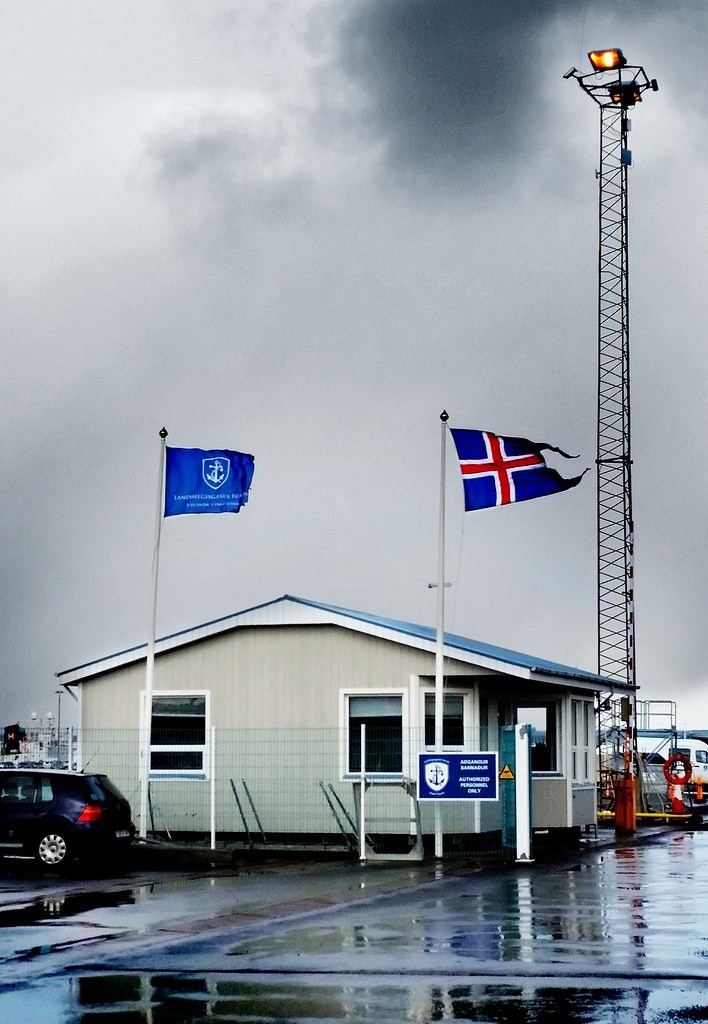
[54,690,64,761]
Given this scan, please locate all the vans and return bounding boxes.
[627,734,708,792]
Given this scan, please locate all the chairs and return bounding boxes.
[1,782,19,802]
[19,786,35,803]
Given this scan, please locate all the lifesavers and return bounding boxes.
[663,755,692,785]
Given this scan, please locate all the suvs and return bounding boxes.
[0,767,136,876]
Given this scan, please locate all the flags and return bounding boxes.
[448,427,592,512]
[163,444,255,517]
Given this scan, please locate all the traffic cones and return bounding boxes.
[665,784,690,814]
